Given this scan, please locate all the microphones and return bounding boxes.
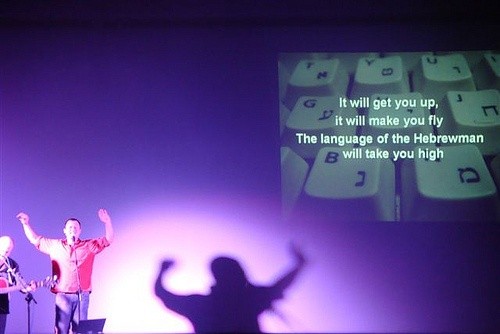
[71,234,76,243]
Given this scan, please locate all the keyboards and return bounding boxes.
[279,51,500,222]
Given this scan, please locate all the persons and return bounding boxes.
[15,209,114,334]
[0,236,37,334]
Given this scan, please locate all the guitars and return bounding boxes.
[0,275,58,296]
